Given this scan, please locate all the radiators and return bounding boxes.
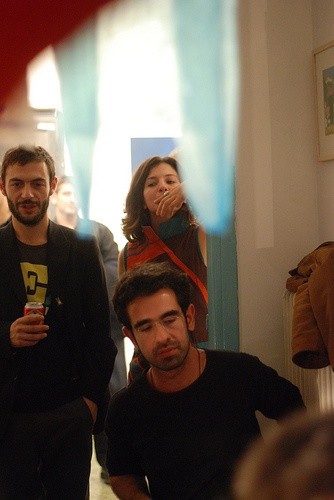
[281,288,334,415]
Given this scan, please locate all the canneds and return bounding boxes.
[24,302,44,334]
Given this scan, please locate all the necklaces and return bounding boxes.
[149,344,203,392]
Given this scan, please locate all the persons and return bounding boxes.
[101,260,308,500]
[43,173,125,486]
[114,153,208,384]
[0,145,120,500]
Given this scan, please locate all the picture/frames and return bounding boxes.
[312,40,334,162]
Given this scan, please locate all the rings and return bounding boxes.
[163,191,169,195]
